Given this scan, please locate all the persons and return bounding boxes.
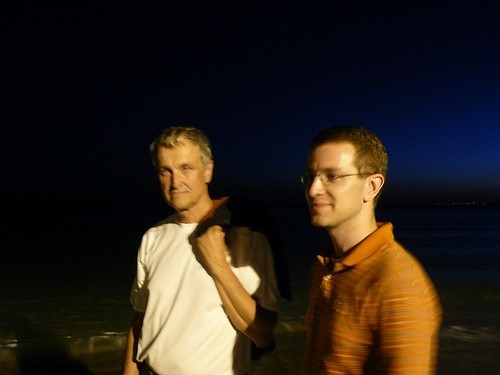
[123,127,279,375]
[300,126,442,375]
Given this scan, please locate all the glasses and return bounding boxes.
[300,171,374,187]
